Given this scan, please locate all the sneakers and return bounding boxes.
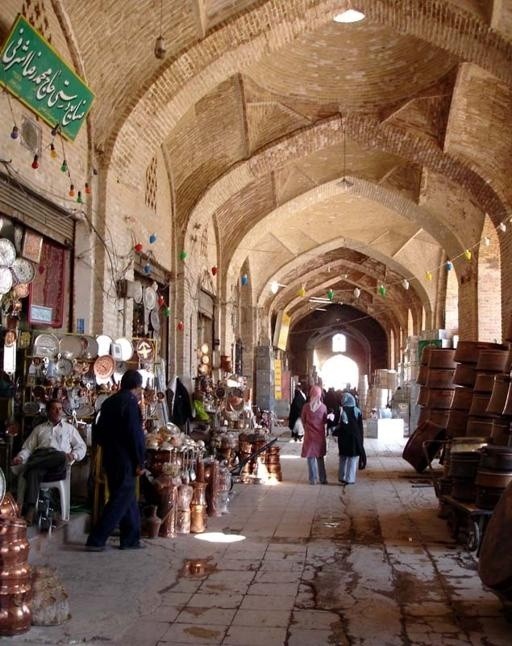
[119,544,147,550]
[10,464,24,475]
[85,546,106,551]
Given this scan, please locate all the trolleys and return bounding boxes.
[423,440,494,551]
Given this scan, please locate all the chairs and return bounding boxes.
[16,462,71,522]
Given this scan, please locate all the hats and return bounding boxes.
[121,369,142,390]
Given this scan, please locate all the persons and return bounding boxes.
[329,392,365,483]
[289,387,308,443]
[301,385,329,483]
[84,367,147,550]
[10,398,88,524]
[294,382,361,441]
